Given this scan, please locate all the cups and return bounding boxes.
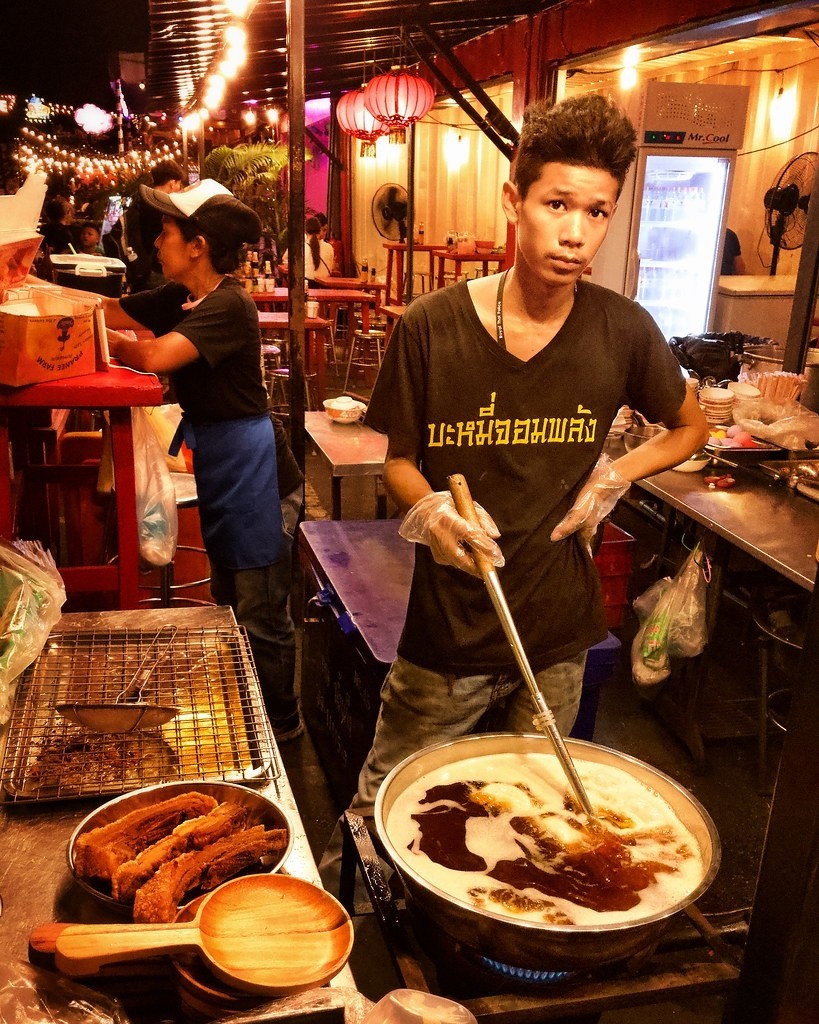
[686,378,699,398]
[699,388,734,424]
[727,382,762,422]
[266,278,275,292]
[306,302,319,319]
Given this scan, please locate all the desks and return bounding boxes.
[307,290,378,335]
[0,330,165,605]
[432,250,504,291]
[256,312,332,413]
[248,285,290,302]
[380,306,412,357]
[313,277,389,317]
[382,242,456,309]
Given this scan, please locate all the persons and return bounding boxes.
[322,93,711,902]
[720,229,745,275]
[42,161,310,745]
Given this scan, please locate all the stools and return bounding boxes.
[342,330,386,406]
[259,343,317,413]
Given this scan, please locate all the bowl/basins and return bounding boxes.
[66,781,292,918]
[474,241,494,254]
[322,396,366,423]
[671,458,710,472]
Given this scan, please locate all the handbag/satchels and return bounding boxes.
[633,575,708,657]
[630,540,706,686]
[130,405,178,566]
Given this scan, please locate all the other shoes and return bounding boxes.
[271,711,302,743]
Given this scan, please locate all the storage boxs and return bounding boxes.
[0,231,96,386]
[594,523,639,628]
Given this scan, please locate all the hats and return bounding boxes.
[86,222,101,235]
[140,179,261,244]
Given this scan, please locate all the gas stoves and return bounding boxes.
[340,790,742,1024]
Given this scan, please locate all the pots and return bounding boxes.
[375,731,721,971]
[729,343,819,413]
[625,413,663,452]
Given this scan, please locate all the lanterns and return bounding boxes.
[335,65,437,158]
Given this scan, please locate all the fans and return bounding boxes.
[764,151,819,277]
[372,183,408,243]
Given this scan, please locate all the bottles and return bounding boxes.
[245,262,252,293]
[371,268,376,283]
[304,280,309,306]
[640,183,705,221]
[448,229,453,253]
[251,252,259,279]
[245,251,252,279]
[635,268,664,301]
[360,258,368,283]
[419,222,424,245]
[265,260,272,278]
[127,247,138,262]
[237,263,244,285]
